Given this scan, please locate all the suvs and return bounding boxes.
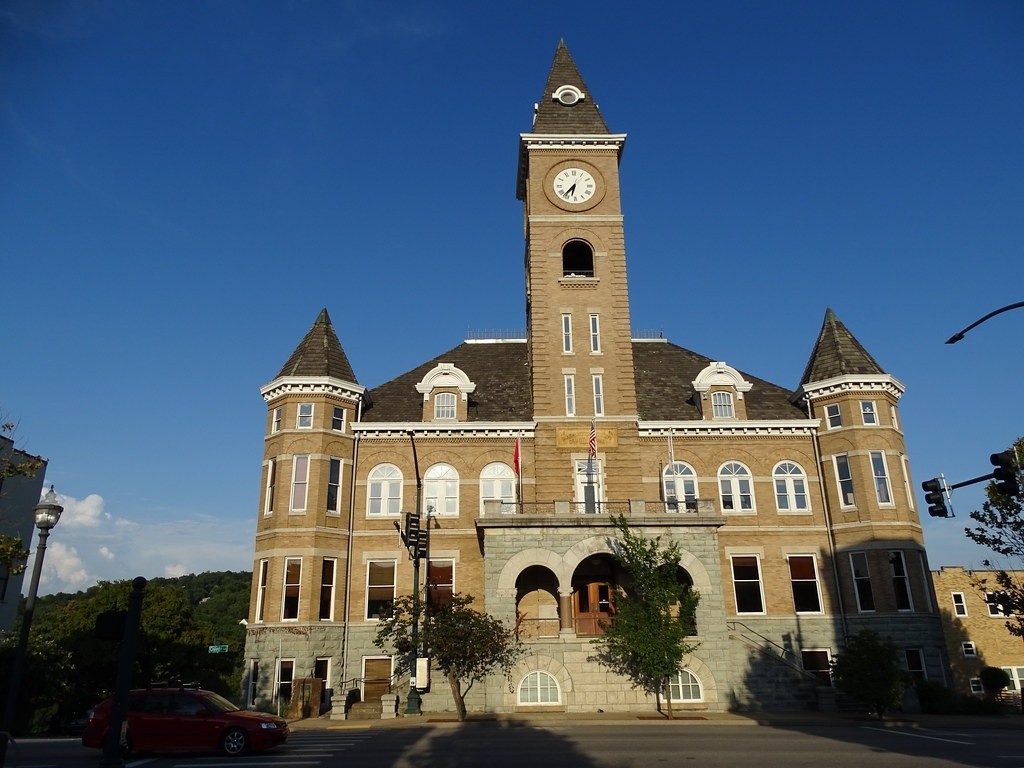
[81,682,290,754]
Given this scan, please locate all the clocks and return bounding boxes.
[553,168,597,203]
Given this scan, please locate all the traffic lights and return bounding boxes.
[404,513,419,547]
[990,451,1019,496]
[922,478,947,518]
[414,530,427,559]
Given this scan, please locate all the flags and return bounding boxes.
[513,439,521,476]
[589,422,596,457]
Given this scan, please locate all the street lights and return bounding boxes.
[406,428,422,715]
[0,485,65,763]
